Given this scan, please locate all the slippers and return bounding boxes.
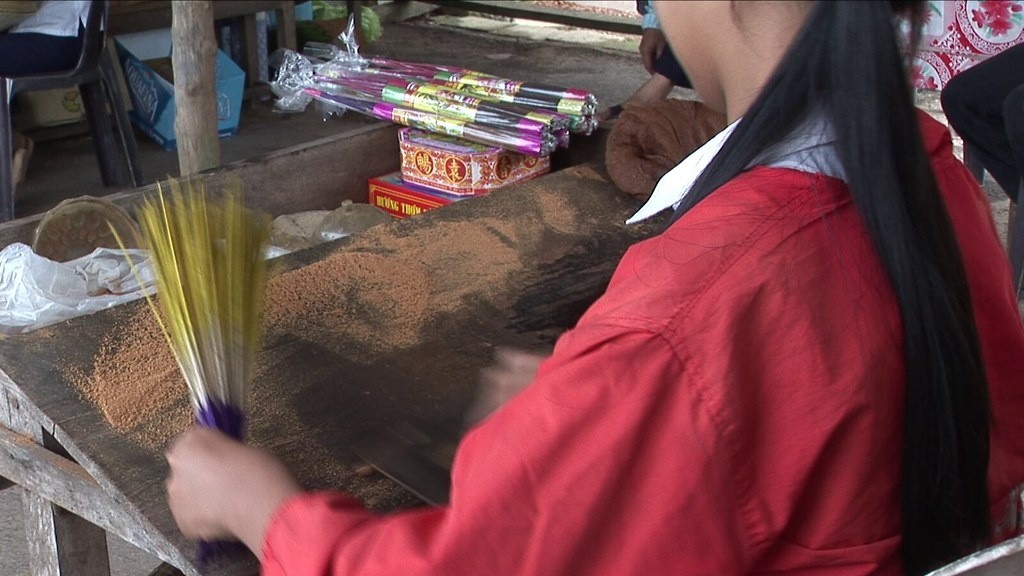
[11,136,34,205]
[590,104,626,131]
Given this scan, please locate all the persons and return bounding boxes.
[1,1,106,187]
[163,0,1024,576]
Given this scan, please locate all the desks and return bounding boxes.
[0,121,613,576]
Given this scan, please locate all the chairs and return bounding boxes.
[0,0,143,221]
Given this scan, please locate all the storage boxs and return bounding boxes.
[16,40,131,133]
[113,22,245,151]
[368,173,470,223]
